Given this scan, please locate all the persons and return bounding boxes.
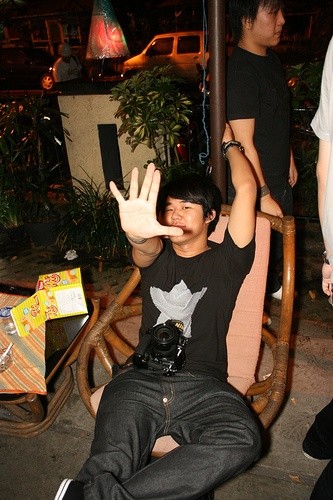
[55,122,264,500]
[300,33,333,500]
[51,44,84,84]
[225,0,298,325]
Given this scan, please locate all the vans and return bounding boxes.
[121,31,209,79]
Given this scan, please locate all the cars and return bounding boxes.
[0,48,59,90]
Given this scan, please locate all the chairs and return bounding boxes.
[74,200,296,456]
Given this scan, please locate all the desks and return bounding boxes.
[0,279,100,438]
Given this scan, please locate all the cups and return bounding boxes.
[0,307,17,334]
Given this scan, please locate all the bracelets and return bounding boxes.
[126,233,147,244]
[322,251,330,265]
[257,184,270,198]
[221,140,244,156]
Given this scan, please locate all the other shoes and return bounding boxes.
[270,287,298,300]
[54,479,84,500]
